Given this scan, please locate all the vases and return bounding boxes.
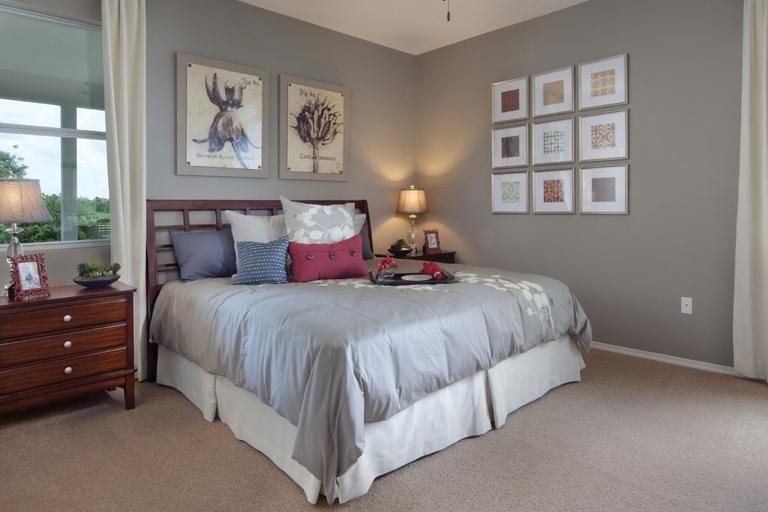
[385,267,395,282]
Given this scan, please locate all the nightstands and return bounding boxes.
[374,249,457,265]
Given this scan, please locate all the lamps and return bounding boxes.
[0,178,54,300]
[396,184,429,256]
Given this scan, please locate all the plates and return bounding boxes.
[400,274,433,282]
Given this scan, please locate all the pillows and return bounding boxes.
[168,196,374,284]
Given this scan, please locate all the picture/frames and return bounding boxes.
[7,252,51,304]
[176,52,271,178]
[423,228,440,256]
[277,73,350,183]
[490,53,630,215]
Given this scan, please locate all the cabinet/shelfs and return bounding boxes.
[0,279,137,420]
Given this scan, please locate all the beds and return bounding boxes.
[147,200,593,508]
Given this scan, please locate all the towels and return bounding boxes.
[421,260,446,280]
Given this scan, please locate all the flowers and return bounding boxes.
[377,255,400,274]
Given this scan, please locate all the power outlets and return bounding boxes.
[680,297,693,314]
